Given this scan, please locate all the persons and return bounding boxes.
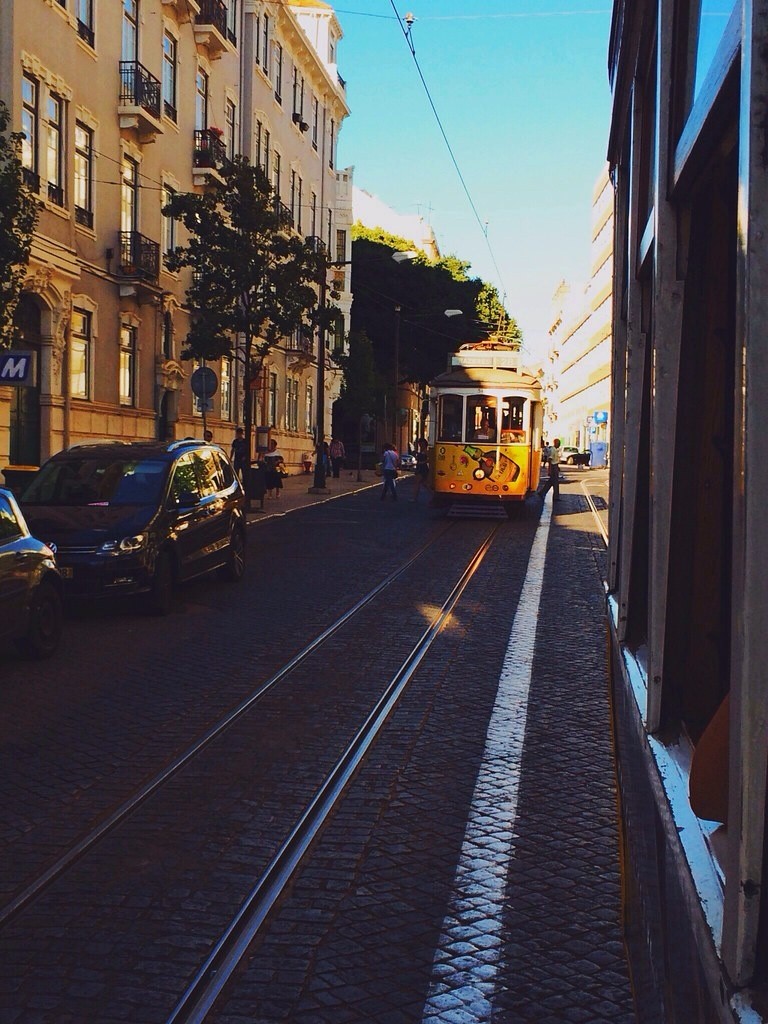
[407,437,433,502]
[204,431,213,442]
[264,439,284,499]
[312,435,345,478]
[380,442,399,500]
[230,428,245,485]
[538,439,561,501]
[473,419,495,441]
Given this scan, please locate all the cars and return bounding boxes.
[0,486,72,655]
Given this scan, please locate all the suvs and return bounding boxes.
[20,437,250,615]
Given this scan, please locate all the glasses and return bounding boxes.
[333,438,336,439]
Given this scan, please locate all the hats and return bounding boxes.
[236,428,244,432]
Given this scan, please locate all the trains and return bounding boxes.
[426,343,544,516]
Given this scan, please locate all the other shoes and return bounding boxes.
[336,475,338,478]
[538,491,544,499]
[276,493,281,498]
[267,497,272,499]
[408,497,416,502]
[553,498,561,502]
[333,475,335,478]
[326,474,331,477]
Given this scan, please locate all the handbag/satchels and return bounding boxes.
[396,472,398,478]
